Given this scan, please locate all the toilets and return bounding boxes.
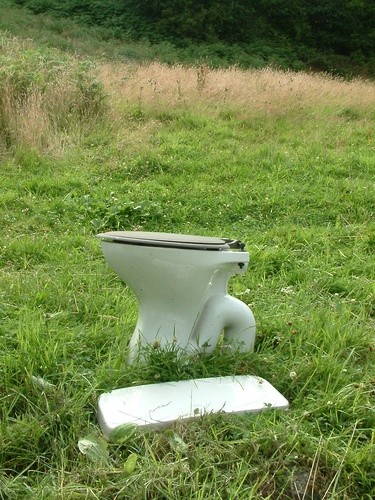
[95,231,256,369]
